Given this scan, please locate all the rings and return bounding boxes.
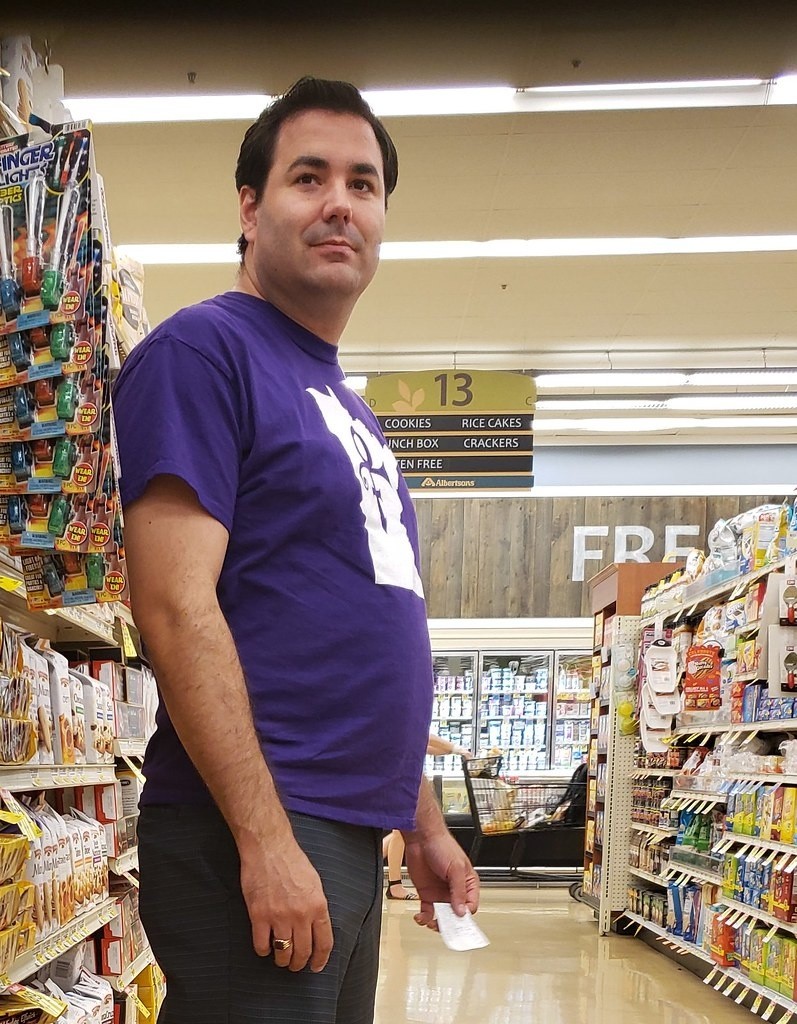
[272,936,294,950]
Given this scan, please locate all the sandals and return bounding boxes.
[385,881,420,901]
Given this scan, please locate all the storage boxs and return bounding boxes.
[0,620,167,1024]
[583,870,593,897]
[592,656,609,688]
[585,820,595,853]
[593,864,601,897]
[592,699,607,729]
[1,33,38,121]
[590,739,598,777]
[594,610,611,647]
[589,780,604,813]
[627,520,797,1003]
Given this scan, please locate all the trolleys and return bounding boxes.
[460,749,587,904]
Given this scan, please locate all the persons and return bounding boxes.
[114,77,480,1024]
[383,733,472,899]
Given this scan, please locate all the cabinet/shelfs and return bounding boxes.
[0,544,155,1024]
[580,552,797,1024]
